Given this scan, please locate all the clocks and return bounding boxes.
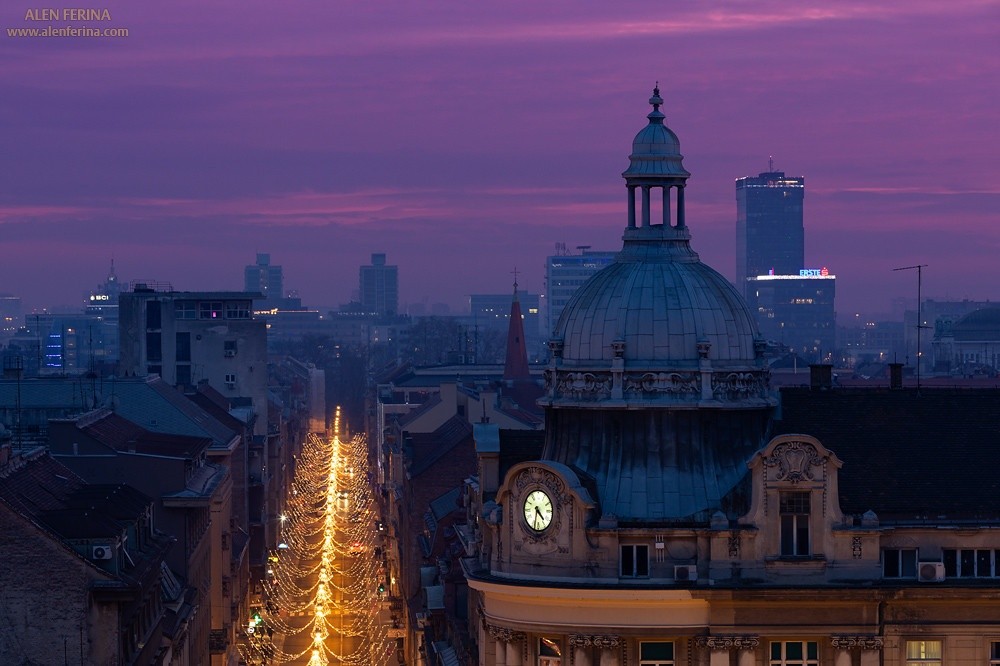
[523,490,552,532]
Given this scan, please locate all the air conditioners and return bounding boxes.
[674,564,697,582]
[91,544,113,561]
[917,561,945,582]
